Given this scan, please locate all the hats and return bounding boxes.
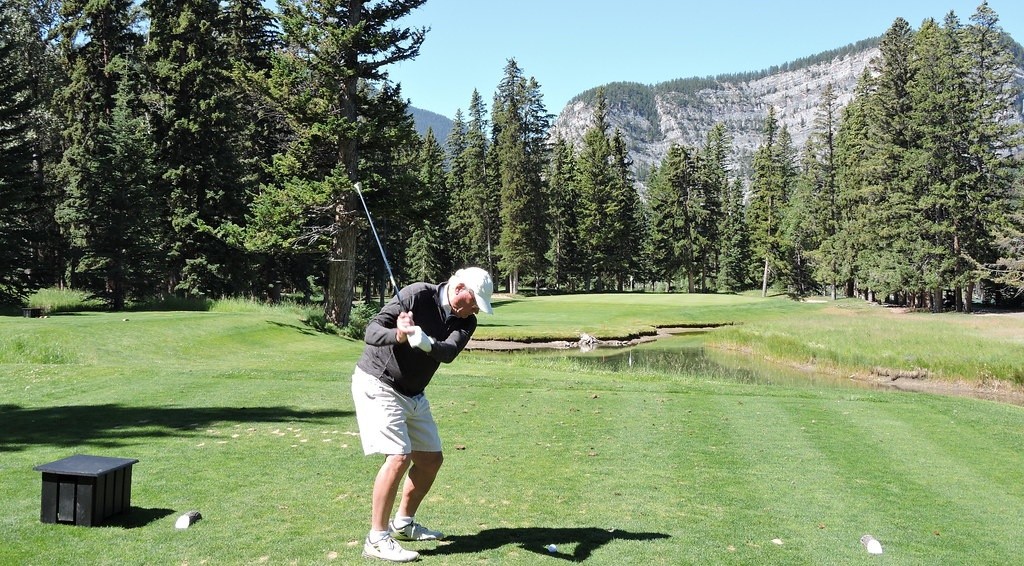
[455,267,494,314]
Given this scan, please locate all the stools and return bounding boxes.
[33,454,140,527]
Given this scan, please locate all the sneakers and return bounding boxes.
[387,519,444,541]
[362,533,420,563]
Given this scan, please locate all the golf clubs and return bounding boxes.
[352,181,409,314]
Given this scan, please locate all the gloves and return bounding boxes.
[406,325,435,352]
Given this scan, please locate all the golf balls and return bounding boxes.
[547,543,557,554]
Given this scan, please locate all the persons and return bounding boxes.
[351,266,494,562]
